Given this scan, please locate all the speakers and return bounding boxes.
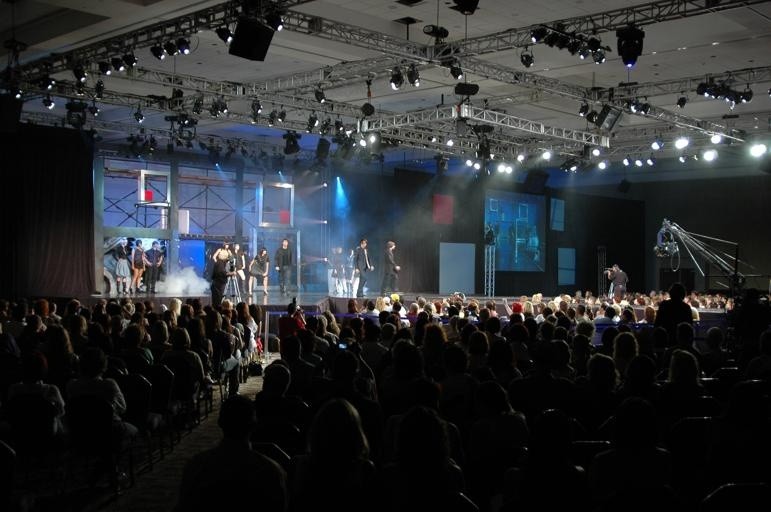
[228,1,276,62]
[618,178,632,192]
[524,170,550,193]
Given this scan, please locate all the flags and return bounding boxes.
[433,194,454,224]
[279,208,290,224]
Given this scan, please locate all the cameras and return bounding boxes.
[229,259,236,272]
[338,342,349,349]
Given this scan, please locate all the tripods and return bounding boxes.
[220,276,242,304]
[607,281,614,298]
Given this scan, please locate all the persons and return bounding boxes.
[341,248,356,293]
[486,220,501,249]
[275,238,294,299]
[213,239,233,262]
[330,246,347,295]
[604,264,629,300]
[230,242,248,295]
[130,240,145,293]
[509,222,516,256]
[115,238,131,294]
[380,240,403,296]
[211,250,237,306]
[248,247,270,296]
[145,241,163,293]
[1,290,771,512]
[353,237,375,297]
